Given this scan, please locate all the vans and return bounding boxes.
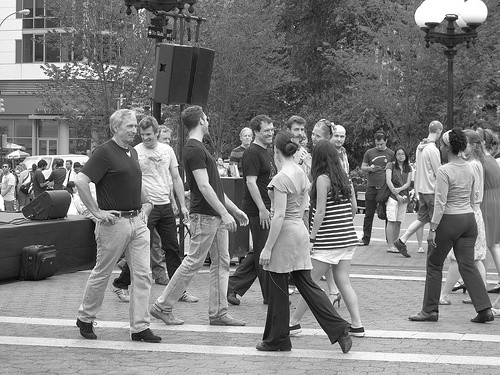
[14,154,89,183]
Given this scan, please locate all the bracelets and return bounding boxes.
[430,228,437,232]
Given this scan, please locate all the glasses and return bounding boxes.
[206,116,210,121]
[2,166,7,168]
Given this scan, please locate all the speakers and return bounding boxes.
[22,190,72,219]
[219,176,250,256]
[151,42,194,106]
[186,46,215,106]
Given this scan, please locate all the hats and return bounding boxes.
[319,118,333,135]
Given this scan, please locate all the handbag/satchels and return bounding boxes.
[20,181,34,194]
[375,162,398,203]
[19,243,57,281]
[64,184,74,195]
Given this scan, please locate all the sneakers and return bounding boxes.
[112,284,130,302]
[178,292,199,302]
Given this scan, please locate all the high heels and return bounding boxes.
[438,281,500,317]
[329,293,341,308]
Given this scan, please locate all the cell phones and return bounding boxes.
[375,166,382,170]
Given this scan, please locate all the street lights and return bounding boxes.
[414,0,489,132]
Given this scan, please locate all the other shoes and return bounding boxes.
[256,341,292,351]
[210,315,247,326]
[471,307,494,322]
[288,324,303,334]
[132,328,161,342]
[227,290,240,305]
[358,239,426,257]
[409,309,438,322]
[337,323,352,353]
[150,306,184,324]
[348,324,365,337]
[76,318,97,339]
[155,274,169,285]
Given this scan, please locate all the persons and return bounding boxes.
[288,139,366,338]
[255,130,353,355]
[383,146,413,252]
[360,131,396,246]
[408,126,495,324]
[149,105,250,326]
[0,156,98,225]
[109,115,500,318]
[74,109,163,344]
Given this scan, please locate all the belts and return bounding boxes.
[111,209,142,218]
